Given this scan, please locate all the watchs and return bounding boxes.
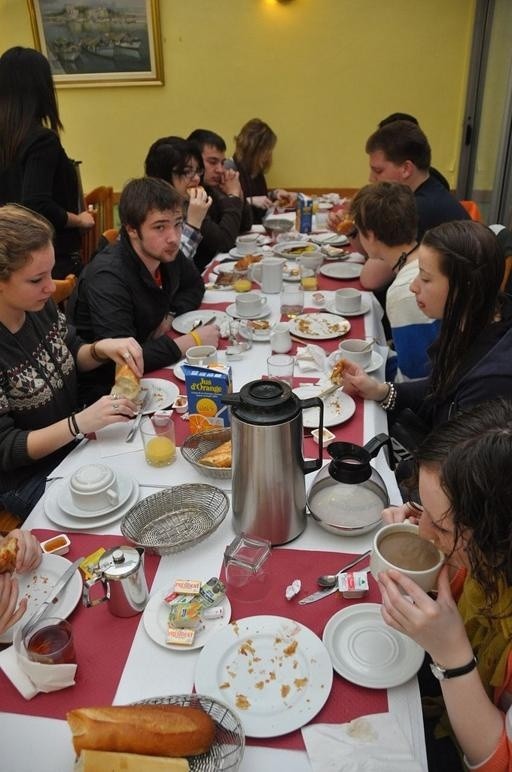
[422,652,482,681]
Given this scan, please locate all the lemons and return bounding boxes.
[188,398,218,433]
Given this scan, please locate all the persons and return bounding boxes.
[326,120,471,245]
[67,176,221,407]
[145,135,214,277]
[374,393,512,772]
[0,46,99,321]
[347,181,442,388]
[379,112,450,191]
[224,118,294,224]
[185,128,253,274]
[331,221,512,504]
[1,529,43,638]
[0,204,145,522]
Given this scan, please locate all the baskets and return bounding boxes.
[180,425,231,479]
[119,483,229,556]
[124,696,245,772]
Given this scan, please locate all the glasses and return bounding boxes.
[176,166,206,178]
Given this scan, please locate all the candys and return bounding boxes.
[162,576,227,645]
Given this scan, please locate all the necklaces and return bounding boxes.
[392,243,421,272]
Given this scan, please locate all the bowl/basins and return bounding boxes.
[370,522,444,593]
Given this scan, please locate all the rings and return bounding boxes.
[121,350,131,359]
[112,403,120,409]
[110,391,121,402]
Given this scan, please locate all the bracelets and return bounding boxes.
[249,195,253,206]
[188,330,202,347]
[67,409,86,447]
[378,380,397,412]
[89,338,107,364]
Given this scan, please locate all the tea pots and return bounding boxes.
[306,431,395,538]
[221,380,324,546]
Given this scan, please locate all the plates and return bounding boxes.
[193,615,333,740]
[173,358,185,383]
[329,348,384,376]
[291,386,356,428]
[143,580,231,651]
[0,553,83,643]
[322,603,425,689]
[133,377,179,416]
[172,192,371,352]
[45,474,139,530]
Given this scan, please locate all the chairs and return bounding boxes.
[78,186,113,266]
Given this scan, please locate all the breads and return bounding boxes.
[185,186,204,195]
[117,365,140,399]
[67,706,215,757]
[78,750,190,771]
[0,539,19,575]
[285,246,312,254]
[249,321,269,329]
[215,272,235,285]
[330,359,346,384]
[337,214,354,233]
[235,254,263,269]
[278,195,295,208]
[200,443,231,465]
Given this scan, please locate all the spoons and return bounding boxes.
[318,549,371,588]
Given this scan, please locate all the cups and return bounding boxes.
[221,530,271,604]
[68,463,118,507]
[339,339,372,370]
[266,353,294,389]
[23,616,75,665]
[140,418,176,469]
[185,345,217,368]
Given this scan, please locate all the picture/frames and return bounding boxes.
[26,0,166,90]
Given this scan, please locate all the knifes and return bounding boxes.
[125,390,153,443]
[22,556,86,635]
[298,566,370,605]
